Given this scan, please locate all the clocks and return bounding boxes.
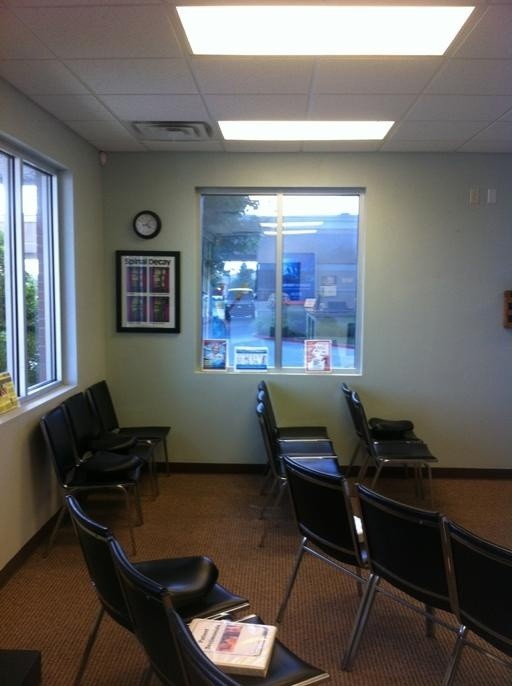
[133,210,162,239]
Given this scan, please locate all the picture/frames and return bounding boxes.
[304,339,332,373]
[201,339,228,372]
[234,347,268,372]
[115,250,181,334]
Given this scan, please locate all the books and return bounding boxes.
[188,619,278,678]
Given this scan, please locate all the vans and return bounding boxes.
[224,288,256,319]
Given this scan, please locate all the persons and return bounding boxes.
[205,345,219,360]
